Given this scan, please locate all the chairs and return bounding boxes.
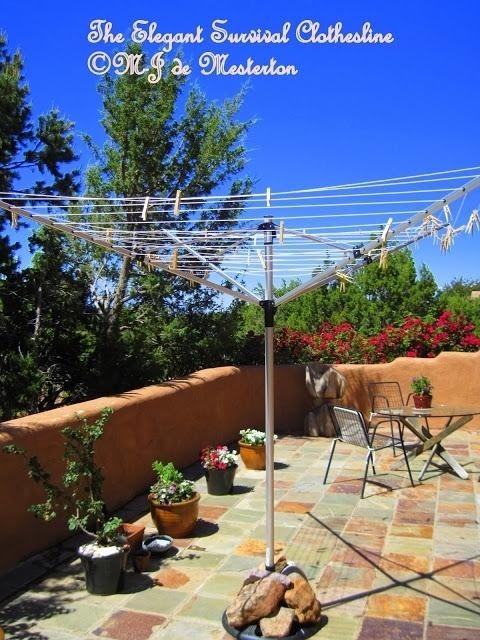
[322,382,480,500]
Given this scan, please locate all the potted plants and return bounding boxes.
[411,372,434,412]
[1,415,128,596]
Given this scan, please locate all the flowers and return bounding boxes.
[150,409,265,506]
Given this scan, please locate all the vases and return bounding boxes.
[149,445,271,537]
